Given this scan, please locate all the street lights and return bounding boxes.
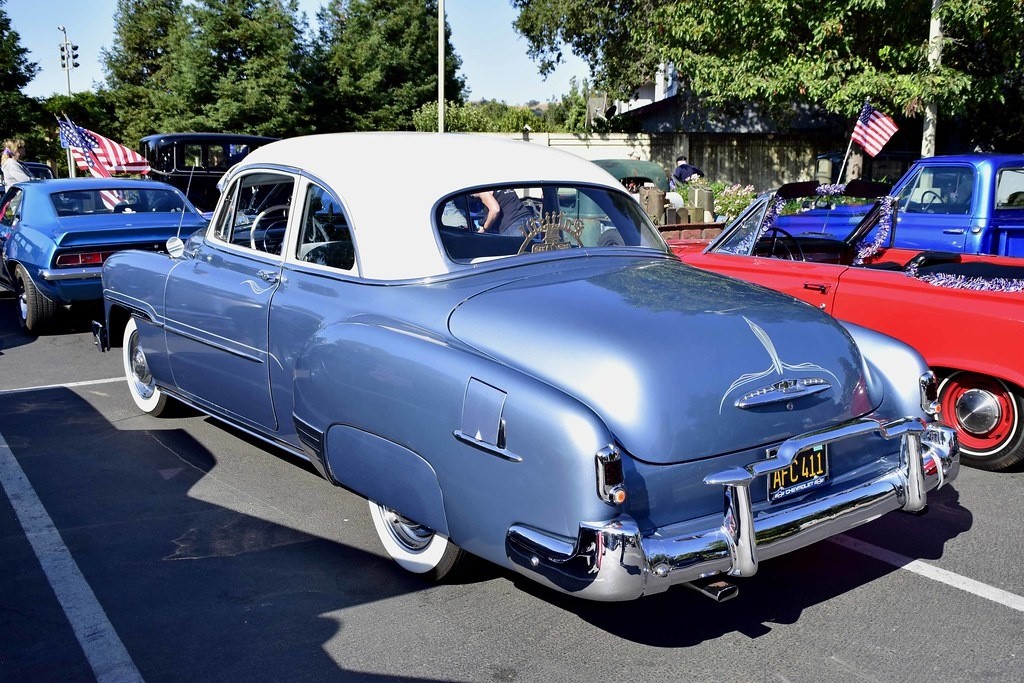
[58,41,80,95]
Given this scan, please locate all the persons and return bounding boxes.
[469,189,538,238]
[951,171,974,206]
[1,138,35,216]
[669,156,704,190]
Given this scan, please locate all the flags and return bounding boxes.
[851,99,898,157]
[56,117,151,174]
[64,113,128,212]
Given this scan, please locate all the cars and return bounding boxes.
[93,130,961,610]
[0,178,212,334]
[0,162,55,202]
[666,179,1024,472]
[137,134,280,212]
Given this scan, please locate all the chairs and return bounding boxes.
[904,248,962,273]
[150,196,177,212]
[857,260,903,271]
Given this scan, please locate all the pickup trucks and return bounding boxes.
[764,156,1024,260]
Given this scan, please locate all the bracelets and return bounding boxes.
[483,225,487,230]
